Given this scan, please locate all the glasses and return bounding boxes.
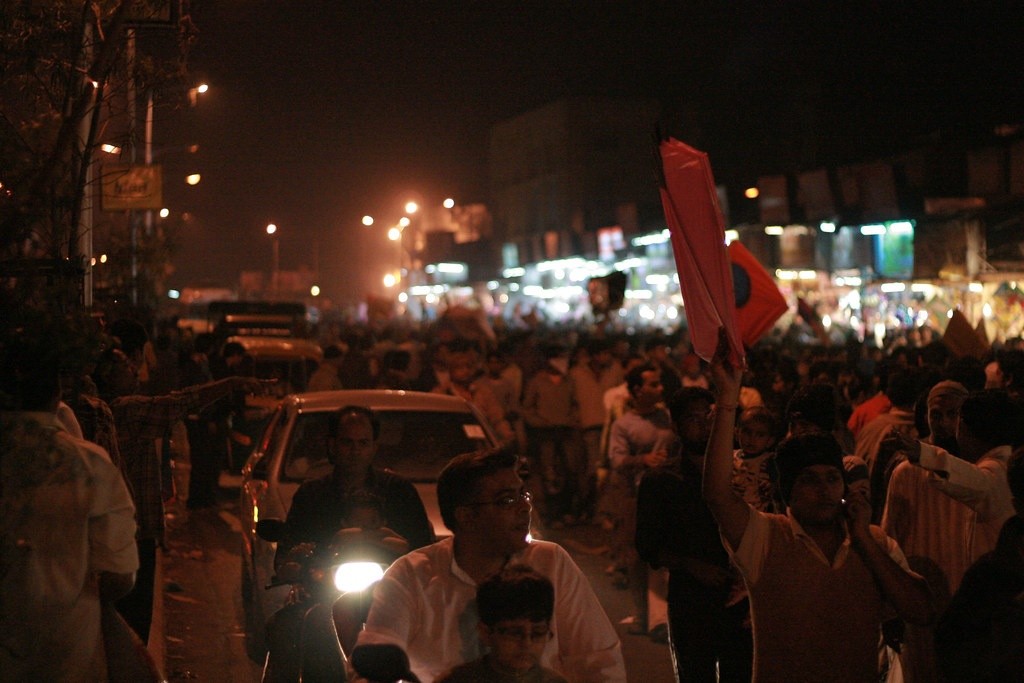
[466,491,533,508]
[490,625,554,643]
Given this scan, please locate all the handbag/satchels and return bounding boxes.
[100,600,166,683]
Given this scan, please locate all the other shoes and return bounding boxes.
[629,614,646,635]
[612,571,628,588]
[650,623,668,644]
[601,519,616,531]
[550,514,579,529]
[606,564,617,575]
[187,500,217,511]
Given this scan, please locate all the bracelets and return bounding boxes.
[708,401,739,411]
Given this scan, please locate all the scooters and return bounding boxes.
[254,514,409,683]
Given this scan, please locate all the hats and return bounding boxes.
[776,430,847,498]
[788,383,835,431]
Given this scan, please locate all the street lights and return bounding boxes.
[142,83,207,166]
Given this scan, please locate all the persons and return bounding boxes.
[260,405,438,591]
[100,284,1022,681]
[0,327,140,683]
[345,444,629,682]
[699,351,947,683]
[433,566,573,683]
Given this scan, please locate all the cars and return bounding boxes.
[216,335,324,427]
[243,388,530,669]
